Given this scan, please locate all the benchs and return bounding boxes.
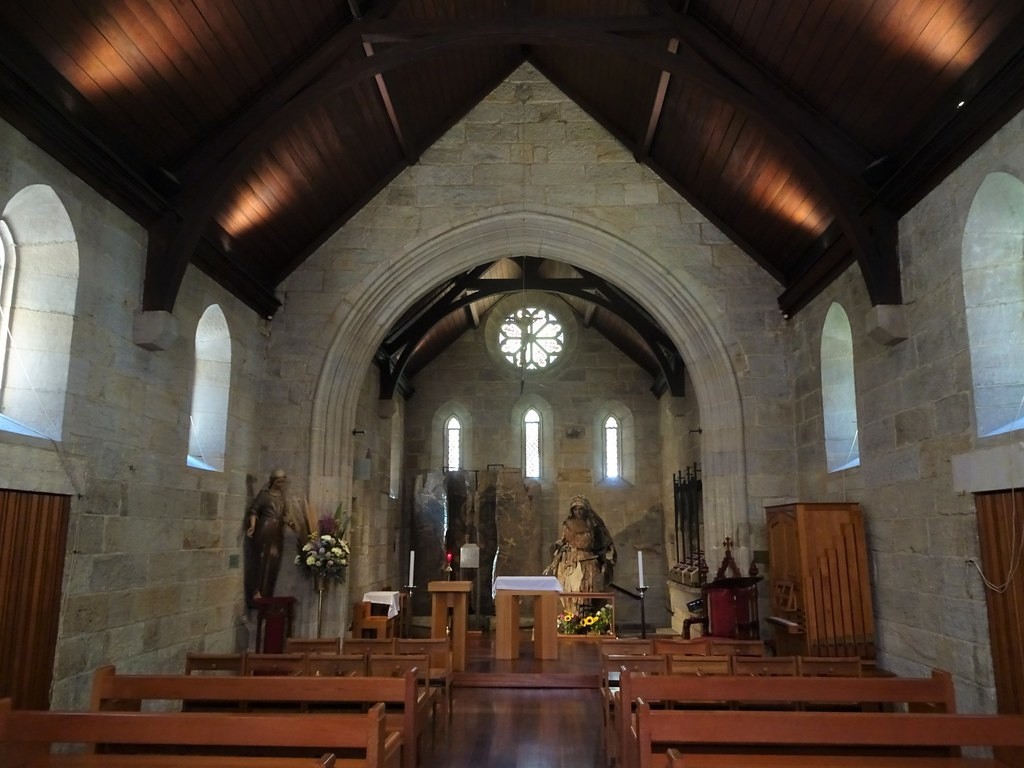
[0,665,433,768]
[614,665,1024,768]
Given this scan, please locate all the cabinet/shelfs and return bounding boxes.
[763,501,881,676]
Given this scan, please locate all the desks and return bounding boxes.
[361,591,401,637]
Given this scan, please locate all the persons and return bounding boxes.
[244,469,296,608]
[542,495,616,620]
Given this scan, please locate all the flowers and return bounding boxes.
[558,602,614,634]
[288,496,355,583]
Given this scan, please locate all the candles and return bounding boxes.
[638,551,644,586]
[409,551,415,587]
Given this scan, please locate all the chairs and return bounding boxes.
[253,596,298,675]
[599,639,862,766]
[682,577,763,658]
[352,602,393,639]
[182,638,455,750]
